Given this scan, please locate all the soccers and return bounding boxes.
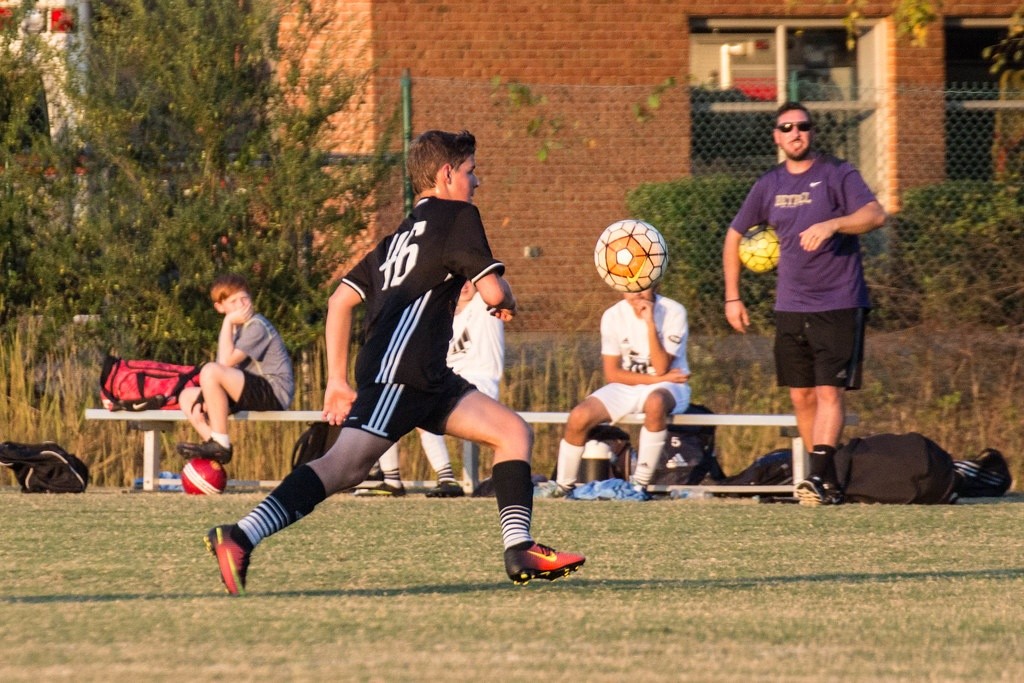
[593,219,667,293]
[738,225,781,274]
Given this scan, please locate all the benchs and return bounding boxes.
[468,412,859,502]
[84,408,471,496]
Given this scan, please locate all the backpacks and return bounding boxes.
[645,402,725,498]
[96,354,203,412]
[0,440,93,495]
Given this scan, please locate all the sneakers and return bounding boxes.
[176,436,233,465]
[425,480,465,498]
[793,472,827,508]
[820,480,845,506]
[359,482,407,498]
[203,522,255,599]
[503,540,587,587]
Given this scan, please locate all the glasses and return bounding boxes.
[774,122,813,132]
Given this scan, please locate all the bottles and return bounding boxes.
[670,491,709,499]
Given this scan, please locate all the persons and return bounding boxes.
[177,274,295,464]
[203,129,585,596]
[549,286,692,500]
[356,280,505,498]
[722,101,888,507]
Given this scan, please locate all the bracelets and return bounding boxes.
[725,298,741,303]
